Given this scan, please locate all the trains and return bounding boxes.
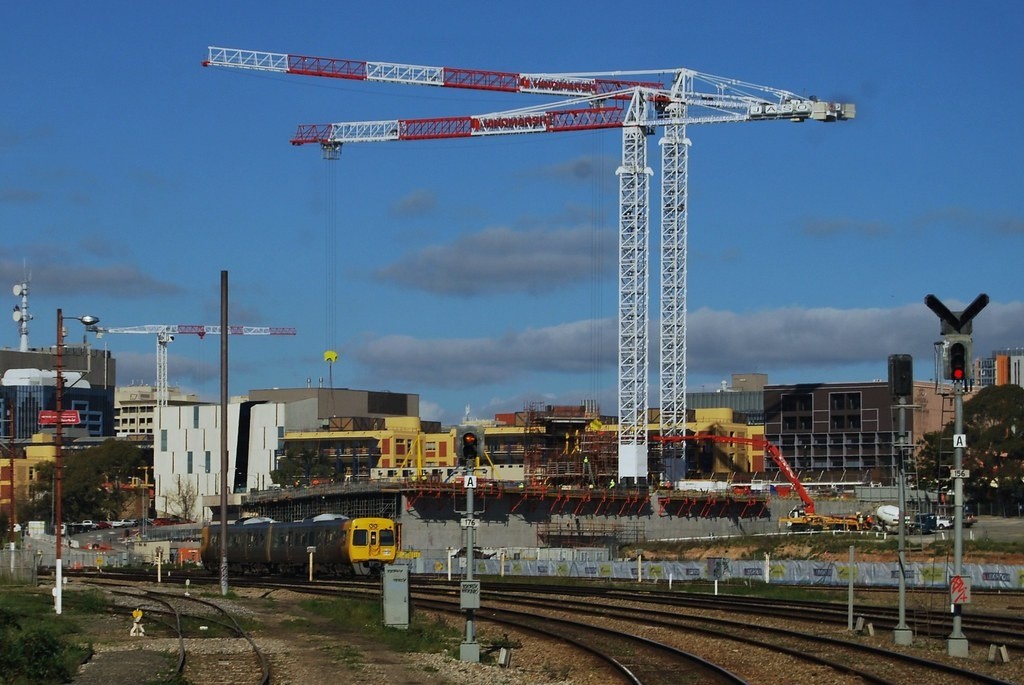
[200,516,399,580]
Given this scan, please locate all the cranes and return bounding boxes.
[287,87,830,485]
[199,43,856,475]
[88,323,296,407]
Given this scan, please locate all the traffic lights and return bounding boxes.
[461,430,478,461]
[947,338,968,383]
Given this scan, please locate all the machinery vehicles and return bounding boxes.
[867,504,914,536]
[649,434,862,532]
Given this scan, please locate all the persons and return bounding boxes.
[858,515,864,531]
[867,515,872,527]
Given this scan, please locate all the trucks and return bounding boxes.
[911,512,936,534]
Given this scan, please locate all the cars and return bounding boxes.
[949,513,977,529]
[932,516,952,530]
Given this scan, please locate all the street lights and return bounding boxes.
[51,308,99,616]
